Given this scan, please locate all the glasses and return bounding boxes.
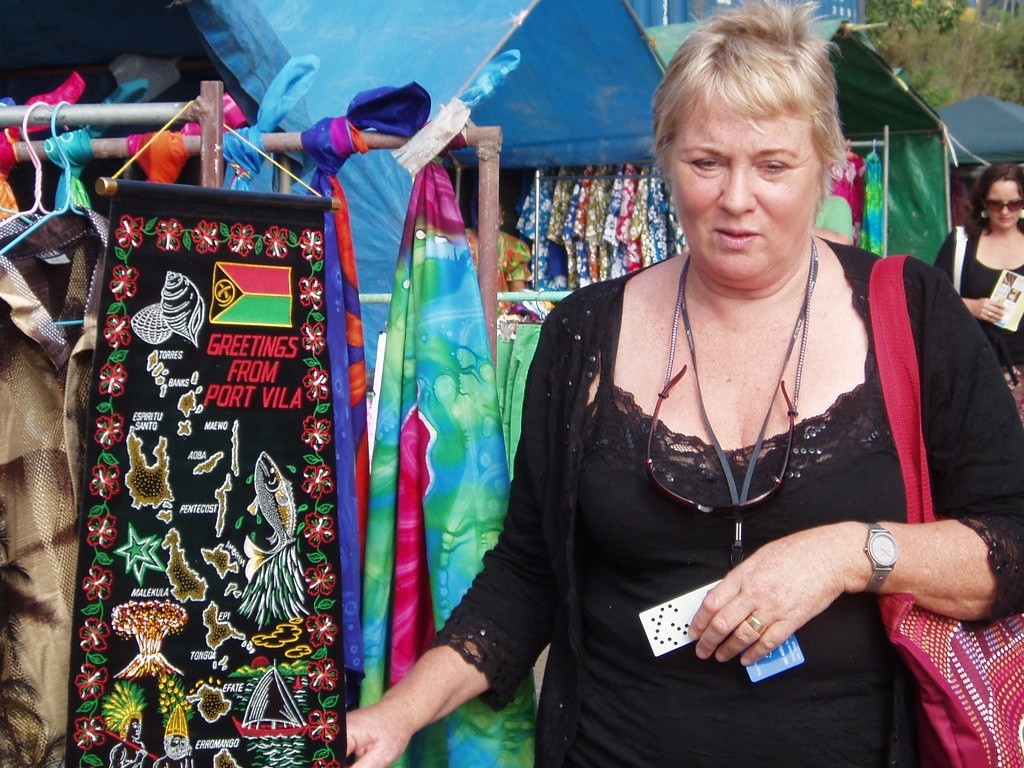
[646,364,795,519]
[981,198,1024,212]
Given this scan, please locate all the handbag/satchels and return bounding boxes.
[869,253,1024,768]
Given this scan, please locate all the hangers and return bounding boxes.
[865,139,881,165]
[0,101,89,327]
[843,139,855,158]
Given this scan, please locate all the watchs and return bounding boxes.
[862,522,898,595]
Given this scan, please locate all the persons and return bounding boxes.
[347,1,1024,768]
[933,161,1024,430]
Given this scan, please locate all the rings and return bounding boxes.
[988,312,991,316]
[746,616,763,633]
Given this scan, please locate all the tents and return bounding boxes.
[936,95,1024,160]
[0,0,681,173]
[643,19,948,265]
